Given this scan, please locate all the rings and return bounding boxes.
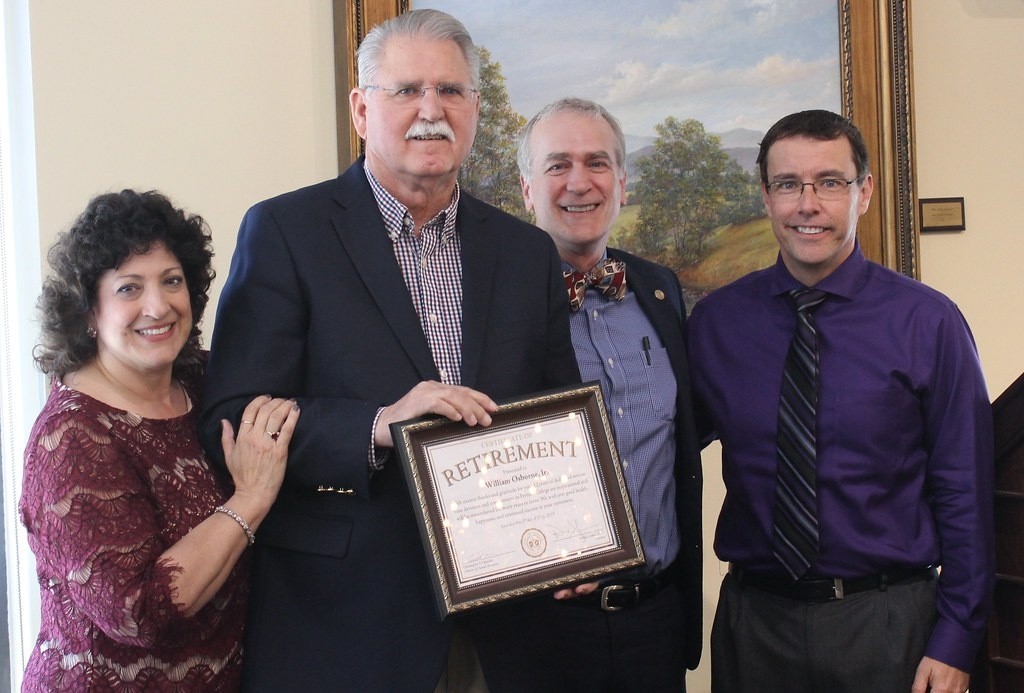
[267,431,279,439]
[240,421,254,425]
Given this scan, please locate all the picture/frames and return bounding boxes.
[332,1,922,321]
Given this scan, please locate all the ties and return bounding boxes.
[767,289,829,590]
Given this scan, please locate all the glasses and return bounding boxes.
[765,176,858,202]
[361,82,482,113]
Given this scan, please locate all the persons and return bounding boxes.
[17,188,301,693]
[684,110,994,693]
[969,371,1023,693]
[514,99,703,693]
[200,7,599,693]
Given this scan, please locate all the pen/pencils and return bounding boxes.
[642,335,653,365]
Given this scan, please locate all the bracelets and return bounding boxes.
[214,507,255,545]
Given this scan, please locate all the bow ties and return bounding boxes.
[561,257,628,312]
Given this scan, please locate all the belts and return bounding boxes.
[727,561,941,605]
[570,583,655,613]
